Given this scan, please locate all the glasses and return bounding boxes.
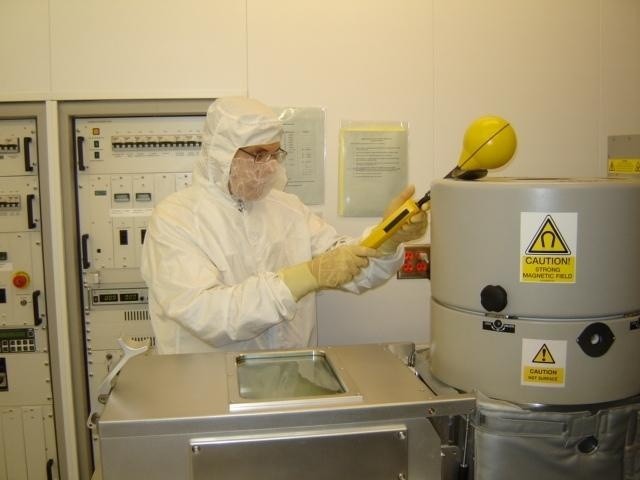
[240,146,288,165]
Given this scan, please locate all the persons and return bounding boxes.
[139,95,430,355]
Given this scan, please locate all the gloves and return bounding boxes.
[277,244,383,302]
[373,185,430,257]
